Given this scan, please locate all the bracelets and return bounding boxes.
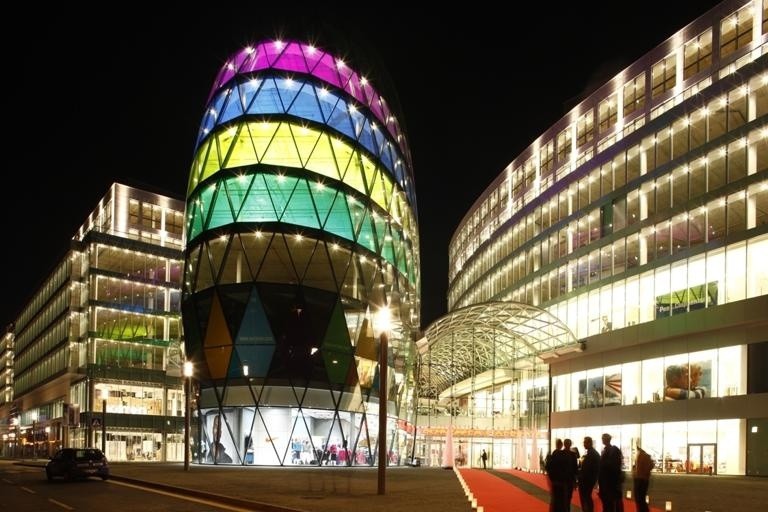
[665,386,671,396]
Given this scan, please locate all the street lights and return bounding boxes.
[373,308,392,494]
[102,390,108,454]
[183,361,192,472]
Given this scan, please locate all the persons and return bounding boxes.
[207,413,233,465]
[481,449,487,469]
[664,362,706,401]
[289,438,313,465]
[577,436,601,512]
[665,366,692,401]
[562,440,577,511]
[547,439,565,512]
[632,438,655,511]
[598,432,624,511]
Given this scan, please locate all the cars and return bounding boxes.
[46,448,109,481]
